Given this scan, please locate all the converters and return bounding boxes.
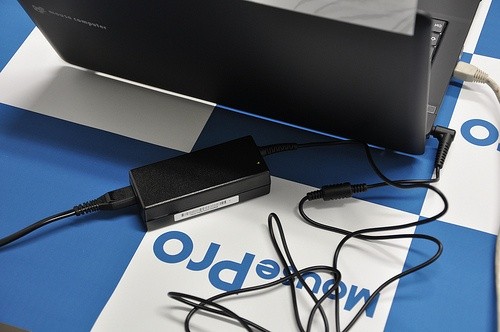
[128,136,271,232]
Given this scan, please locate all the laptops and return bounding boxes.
[17,0,480,155]
